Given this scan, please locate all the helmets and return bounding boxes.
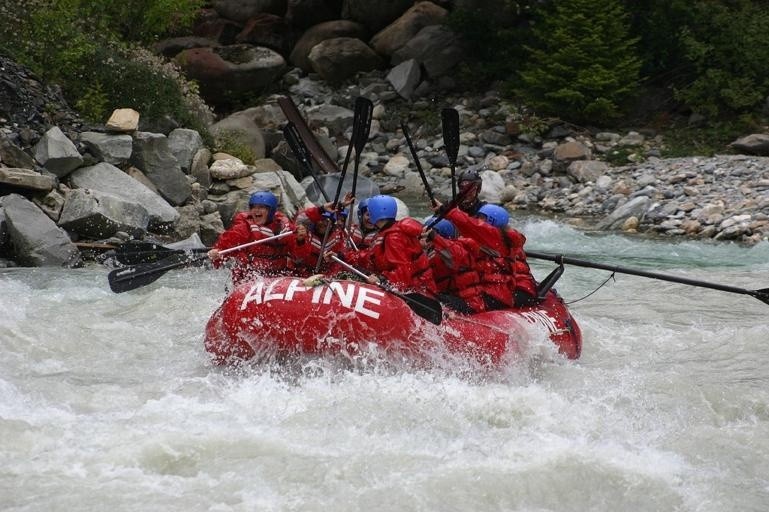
[312,195,409,226]
[478,203,510,230]
[458,169,482,195]
[249,191,278,220]
[426,216,455,244]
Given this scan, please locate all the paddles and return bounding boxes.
[347,96,373,242]
[426,182,475,232]
[283,120,331,203]
[441,107,458,203]
[526,252,769,305]
[108,229,299,292]
[398,120,437,212]
[312,98,361,274]
[311,240,442,326]
[116,240,214,265]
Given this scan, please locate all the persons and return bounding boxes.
[206,169,537,313]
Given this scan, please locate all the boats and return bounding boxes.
[203,277,582,368]
